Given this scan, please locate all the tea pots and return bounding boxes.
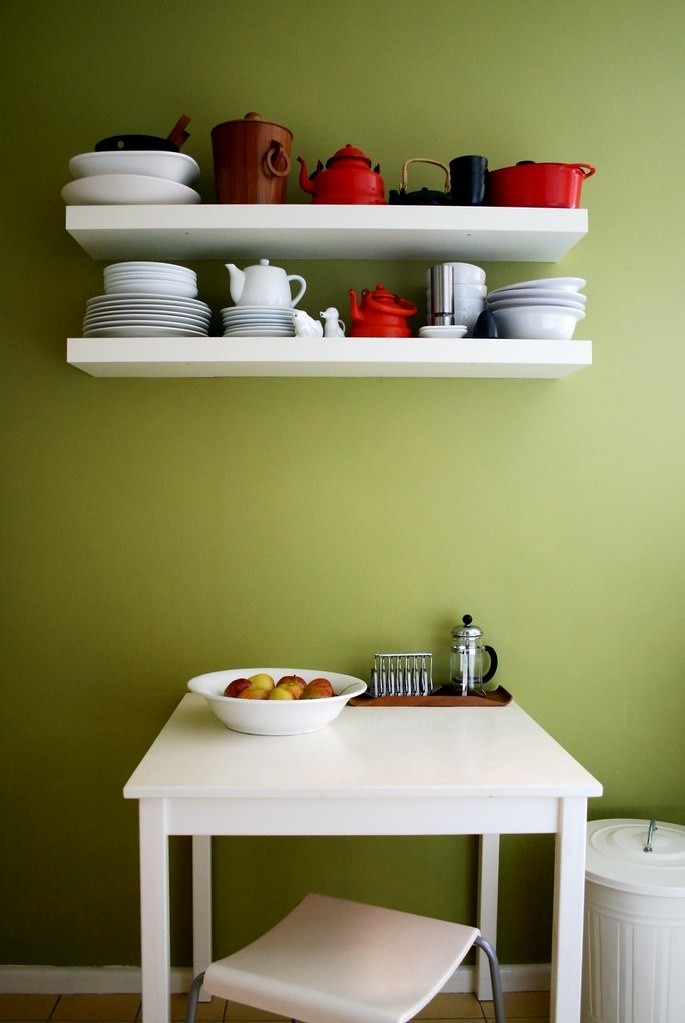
[296,143,389,205]
[346,282,418,338]
[387,157,450,206]
[223,258,307,306]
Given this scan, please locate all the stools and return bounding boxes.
[188,890,506,1023]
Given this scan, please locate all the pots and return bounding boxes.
[488,160,596,207]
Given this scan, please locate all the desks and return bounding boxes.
[124,695,604,1023]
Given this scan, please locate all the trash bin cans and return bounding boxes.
[583,818,685,1022]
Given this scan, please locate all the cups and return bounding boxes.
[450,155,488,205]
[431,264,454,325]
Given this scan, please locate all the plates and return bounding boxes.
[220,306,303,337]
[60,150,203,204]
[80,260,212,338]
[419,325,468,338]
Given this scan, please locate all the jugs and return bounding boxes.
[447,614,498,695]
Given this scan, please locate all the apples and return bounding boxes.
[224,673,334,700]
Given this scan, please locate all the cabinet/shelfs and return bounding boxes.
[61,205,593,379]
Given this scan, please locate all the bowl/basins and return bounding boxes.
[425,262,488,329]
[94,133,180,153]
[487,276,587,339]
[186,667,368,735]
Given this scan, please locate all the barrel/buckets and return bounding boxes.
[209,119,294,204]
